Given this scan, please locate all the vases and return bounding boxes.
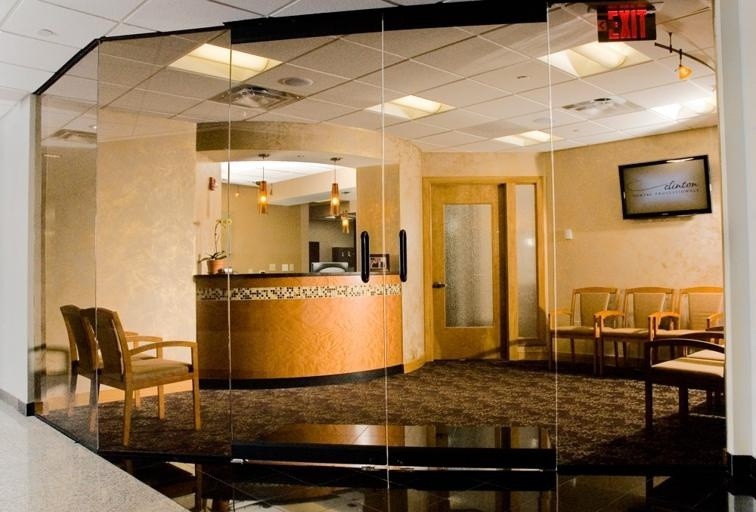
[206,259,225,276]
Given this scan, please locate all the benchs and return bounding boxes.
[230,420,556,512]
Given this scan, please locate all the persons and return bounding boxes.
[371,258,383,267]
[371,258,376,266]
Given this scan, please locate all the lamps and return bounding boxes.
[337,201,352,236]
[329,160,340,218]
[256,166,270,215]
[655,29,716,92]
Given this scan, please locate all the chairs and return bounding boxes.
[60,302,163,432]
[653,284,724,359]
[683,325,726,359]
[645,337,725,442]
[543,286,621,367]
[597,286,675,376]
[82,307,201,445]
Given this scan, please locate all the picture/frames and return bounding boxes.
[371,253,391,272]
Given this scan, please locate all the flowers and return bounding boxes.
[196,214,233,260]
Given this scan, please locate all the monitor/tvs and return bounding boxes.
[619,154,711,219]
[311,262,349,272]
